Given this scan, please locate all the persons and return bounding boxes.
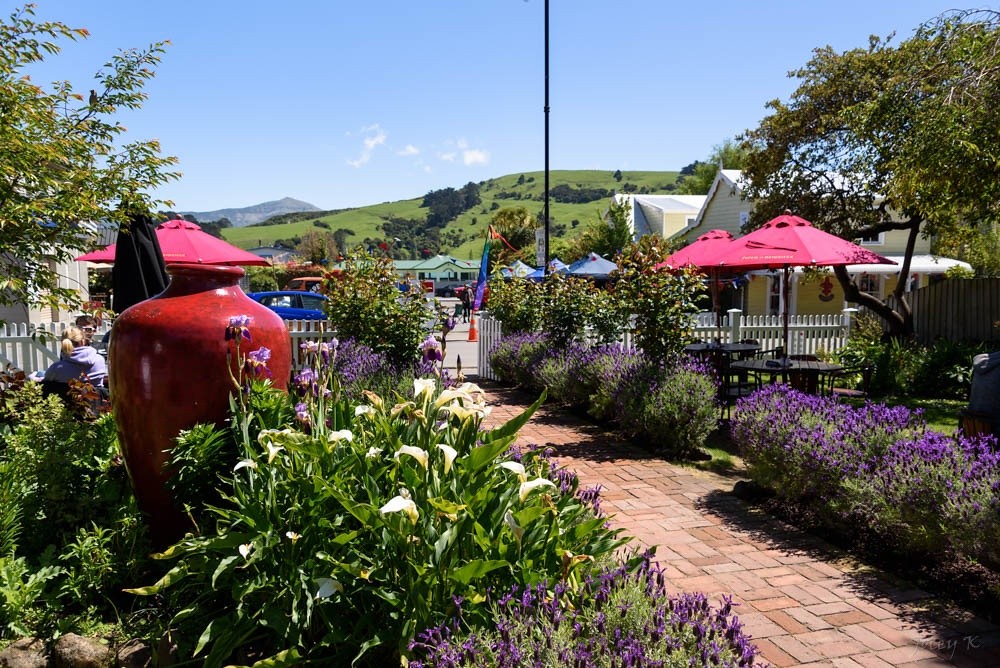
[45,315,118,411]
[461,284,473,323]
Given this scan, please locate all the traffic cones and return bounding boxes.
[466,314,480,342]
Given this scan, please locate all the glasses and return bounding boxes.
[77,325,96,333]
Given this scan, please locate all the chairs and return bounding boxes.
[685,338,876,408]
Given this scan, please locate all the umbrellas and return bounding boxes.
[641,209,898,359]
[73,213,273,266]
[487,251,628,283]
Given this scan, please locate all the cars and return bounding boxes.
[283,277,324,291]
[434,284,461,298]
[454,280,478,298]
[247,294,332,318]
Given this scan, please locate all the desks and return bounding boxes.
[686,343,762,377]
[728,358,844,394]
